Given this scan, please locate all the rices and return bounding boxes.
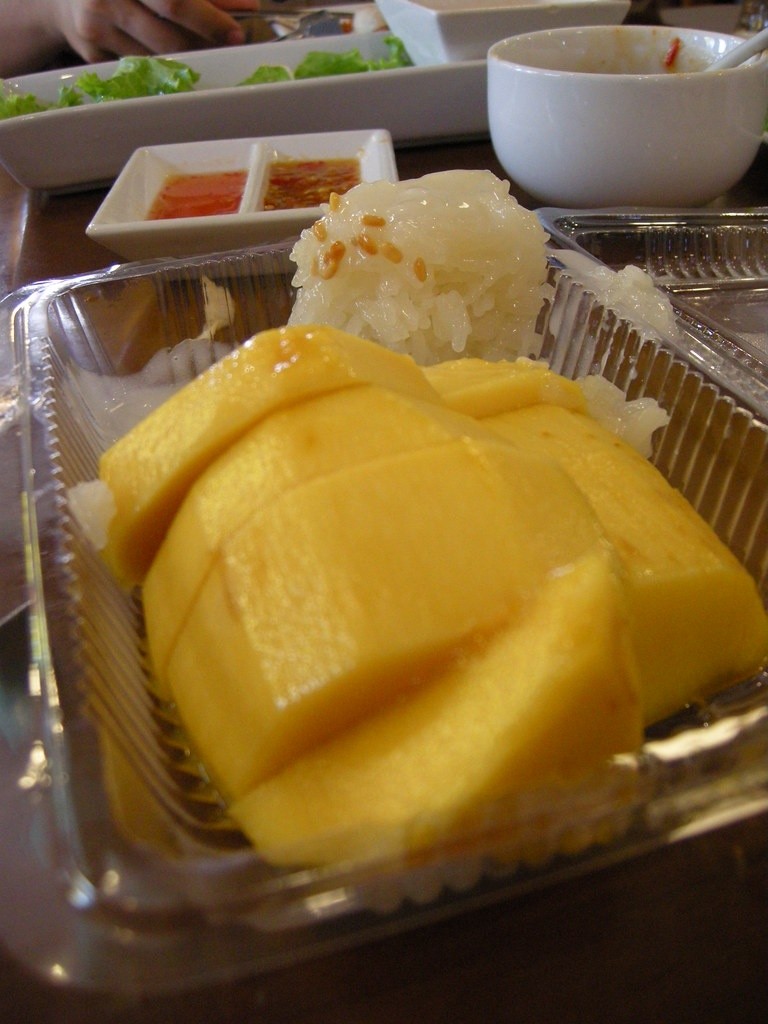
[281,168,678,467]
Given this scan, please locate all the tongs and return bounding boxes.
[268,10,342,42]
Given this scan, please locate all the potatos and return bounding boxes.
[89,319,768,915]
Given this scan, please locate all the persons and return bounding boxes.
[0,0,245,79]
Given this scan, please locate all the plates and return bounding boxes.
[271,4,380,42]
[83,128,401,284]
[0,31,493,198]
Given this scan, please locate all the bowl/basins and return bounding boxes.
[656,0,742,35]
[374,0,633,67]
[486,25,768,208]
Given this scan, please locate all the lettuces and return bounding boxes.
[0,37,417,124]
